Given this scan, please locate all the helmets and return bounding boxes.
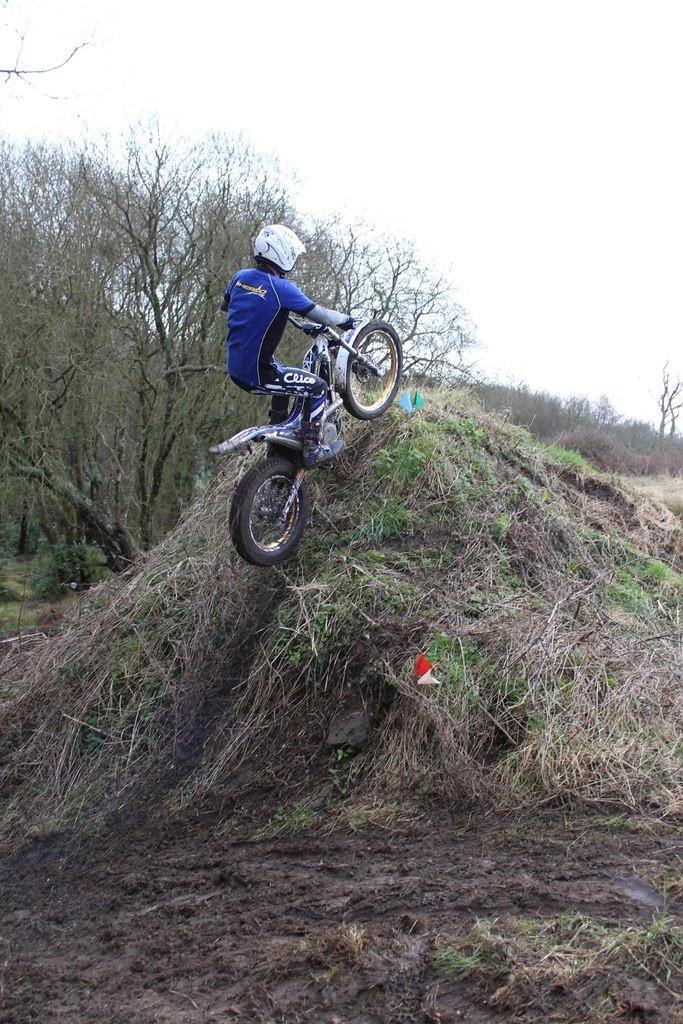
[253,224,306,273]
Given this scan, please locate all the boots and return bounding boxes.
[268,410,288,424]
[299,422,345,471]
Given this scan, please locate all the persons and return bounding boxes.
[220,224,357,470]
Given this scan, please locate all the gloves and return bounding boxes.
[336,314,356,331]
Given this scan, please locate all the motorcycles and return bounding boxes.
[206,314,405,570]
